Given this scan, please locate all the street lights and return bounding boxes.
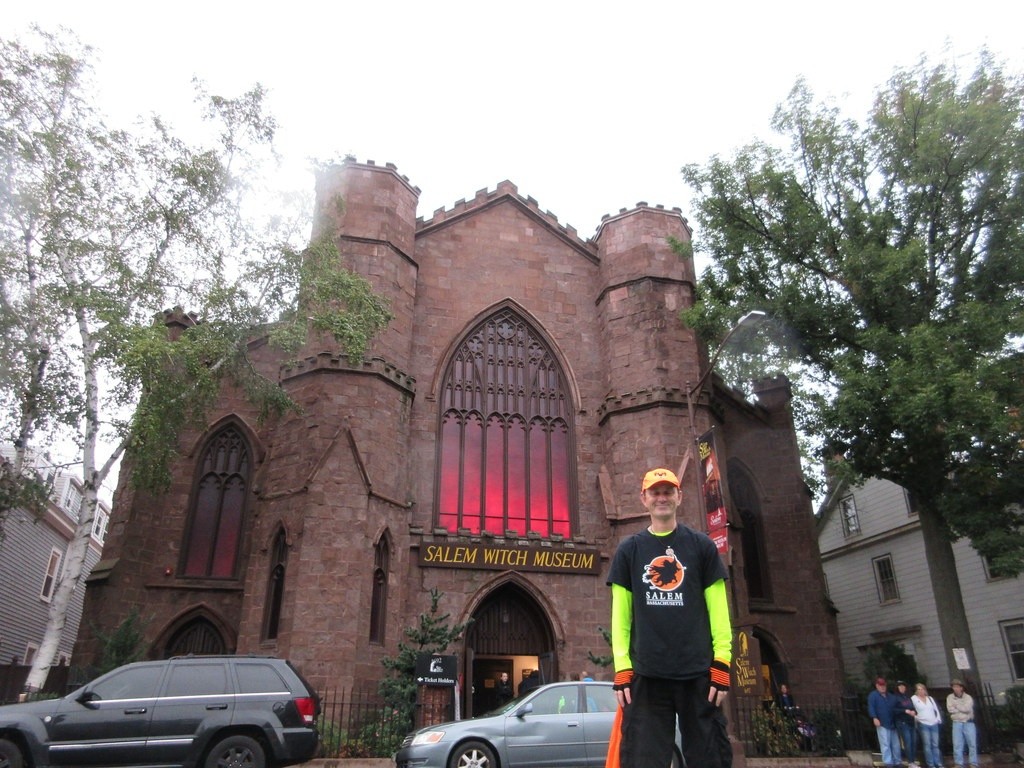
[686,310,767,535]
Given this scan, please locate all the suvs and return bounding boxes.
[0,652,323,768]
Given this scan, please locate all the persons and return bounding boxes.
[607,467,733,768]
[518,670,539,695]
[706,483,719,513]
[946,679,979,768]
[570,671,594,681]
[868,677,944,768]
[776,684,799,711]
[495,673,513,707]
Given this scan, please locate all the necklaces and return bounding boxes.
[651,526,678,556]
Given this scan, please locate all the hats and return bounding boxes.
[875,677,886,685]
[642,468,680,491]
[950,678,964,686]
[895,680,909,687]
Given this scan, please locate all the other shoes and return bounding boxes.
[886,763,894,768]
[908,763,921,768]
[969,764,977,768]
[935,763,944,768]
[896,763,908,768]
[952,764,964,768]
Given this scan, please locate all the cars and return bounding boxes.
[393,679,683,768]
